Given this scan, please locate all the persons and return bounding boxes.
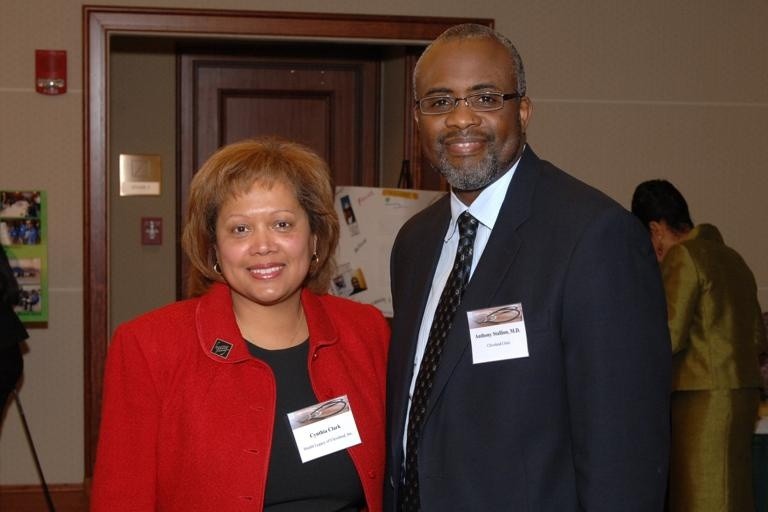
[382,23,673,511]
[91,136,391,512]
[632,178,761,512]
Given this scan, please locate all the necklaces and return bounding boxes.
[287,309,305,348]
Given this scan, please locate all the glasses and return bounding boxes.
[416,93,520,114]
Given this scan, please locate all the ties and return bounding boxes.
[405,212,478,511]
